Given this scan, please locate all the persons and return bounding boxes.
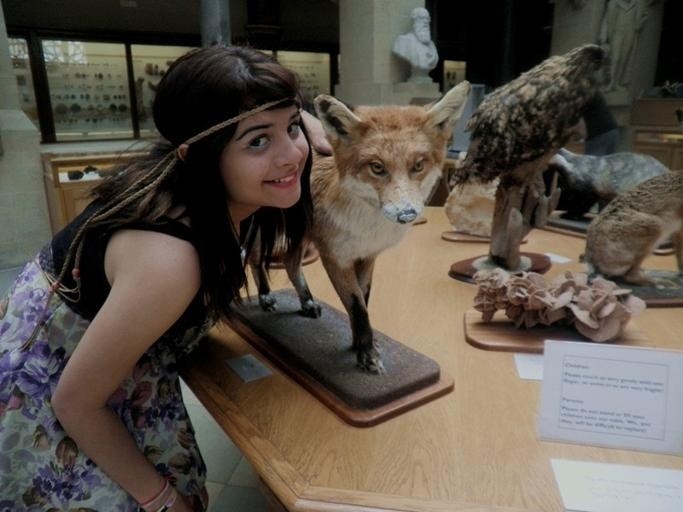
[0,44,336,512]
[393,6,438,71]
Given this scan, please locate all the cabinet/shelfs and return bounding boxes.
[632,127,683,170]
[5,23,336,142]
[39,149,150,239]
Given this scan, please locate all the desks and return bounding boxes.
[180,204,682,512]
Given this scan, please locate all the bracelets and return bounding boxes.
[141,480,179,512]
